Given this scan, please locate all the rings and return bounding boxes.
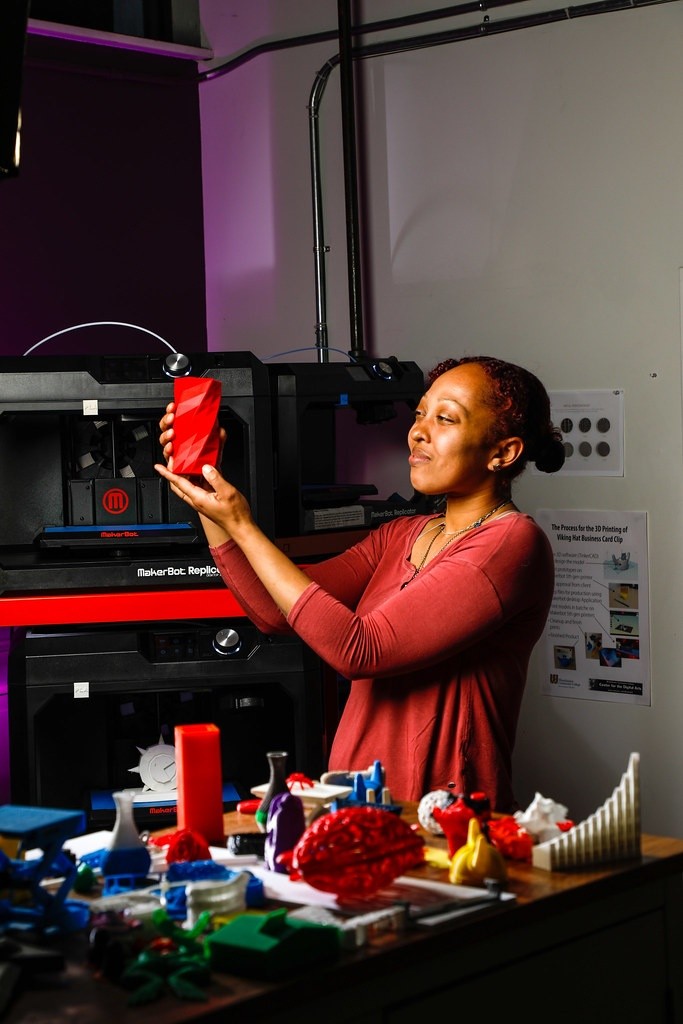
[182,493,186,499]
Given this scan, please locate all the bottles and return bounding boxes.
[101,792,151,883]
[255,752,291,833]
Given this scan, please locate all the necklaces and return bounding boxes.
[398,494,509,591]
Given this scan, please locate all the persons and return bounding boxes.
[150,355,569,823]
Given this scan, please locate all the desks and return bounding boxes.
[0,810,683,1024]
